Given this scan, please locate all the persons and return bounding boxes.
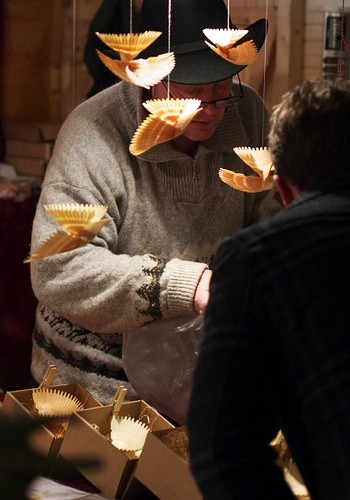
[185,79,350,500]
[29,0,280,426]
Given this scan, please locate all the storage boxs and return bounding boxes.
[4,118,58,193]
[3,374,210,500]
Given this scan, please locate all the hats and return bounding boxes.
[105,0,269,85]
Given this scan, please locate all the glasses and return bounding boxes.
[161,73,244,109]
